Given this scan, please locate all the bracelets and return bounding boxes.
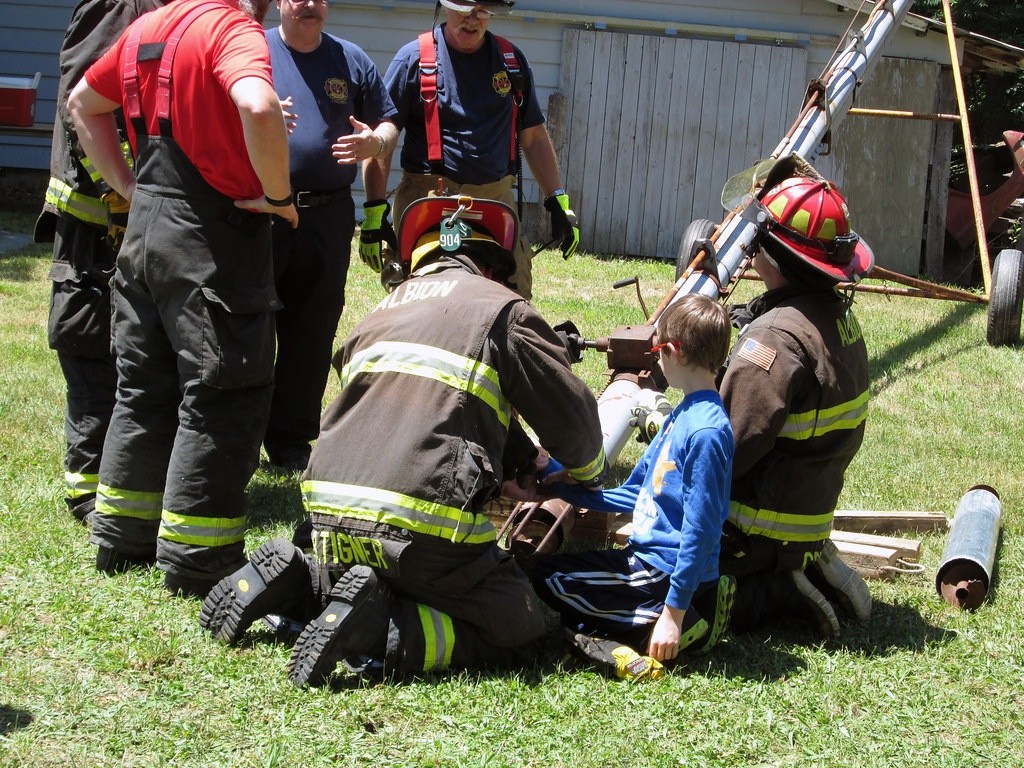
[544,188,566,202]
[264,187,293,207]
[374,135,384,159]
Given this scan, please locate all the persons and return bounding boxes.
[31,0,172,523]
[364,0,579,303]
[198,141,868,692]
[262,0,399,480]
[67,0,298,593]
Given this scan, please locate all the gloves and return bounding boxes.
[573,633,664,685]
[544,194,579,261]
[359,200,398,273]
[100,188,131,252]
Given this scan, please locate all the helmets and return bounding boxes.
[429,0,516,44]
[396,193,520,289]
[721,151,875,282]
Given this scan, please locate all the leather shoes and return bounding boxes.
[287,564,394,690]
[200,539,309,648]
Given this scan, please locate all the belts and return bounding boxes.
[292,186,350,207]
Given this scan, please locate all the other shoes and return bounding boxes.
[783,570,840,647]
[165,557,250,599]
[96,545,156,574]
[293,450,312,470]
[81,510,95,527]
[804,538,872,621]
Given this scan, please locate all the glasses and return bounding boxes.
[649,341,684,360]
[293,0,329,4]
[456,10,493,20]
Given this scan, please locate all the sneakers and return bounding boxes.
[689,574,737,657]
[636,604,708,657]
